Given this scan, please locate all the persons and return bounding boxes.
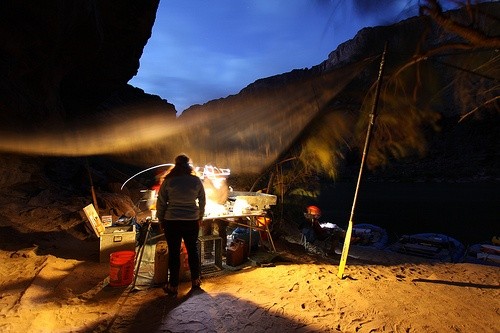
[156,155,206,294]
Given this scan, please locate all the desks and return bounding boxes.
[133,210,277,288]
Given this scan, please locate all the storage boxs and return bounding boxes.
[83,203,136,264]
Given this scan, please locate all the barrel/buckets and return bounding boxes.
[110,251,135,286]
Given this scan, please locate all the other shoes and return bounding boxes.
[165,283,180,295]
[191,278,201,289]
